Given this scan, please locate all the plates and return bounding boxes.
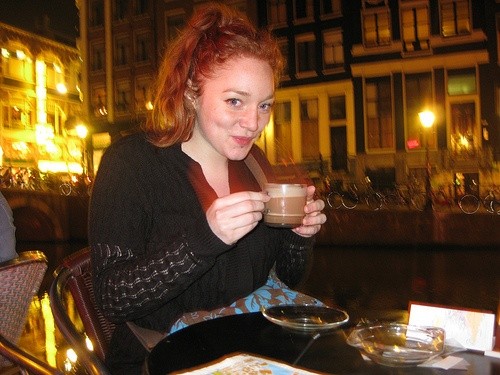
[262,305,349,336]
[345,323,445,366]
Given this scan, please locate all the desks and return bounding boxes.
[145,312,500,375]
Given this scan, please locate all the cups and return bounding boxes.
[259,183,306,228]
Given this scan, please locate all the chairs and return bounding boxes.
[0,247,116,375]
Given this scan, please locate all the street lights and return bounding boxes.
[415,109,439,216]
[76,124,92,196]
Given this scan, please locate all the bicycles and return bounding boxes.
[325,177,500,213]
[0,166,100,196]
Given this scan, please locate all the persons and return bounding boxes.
[89,2,328,375]
